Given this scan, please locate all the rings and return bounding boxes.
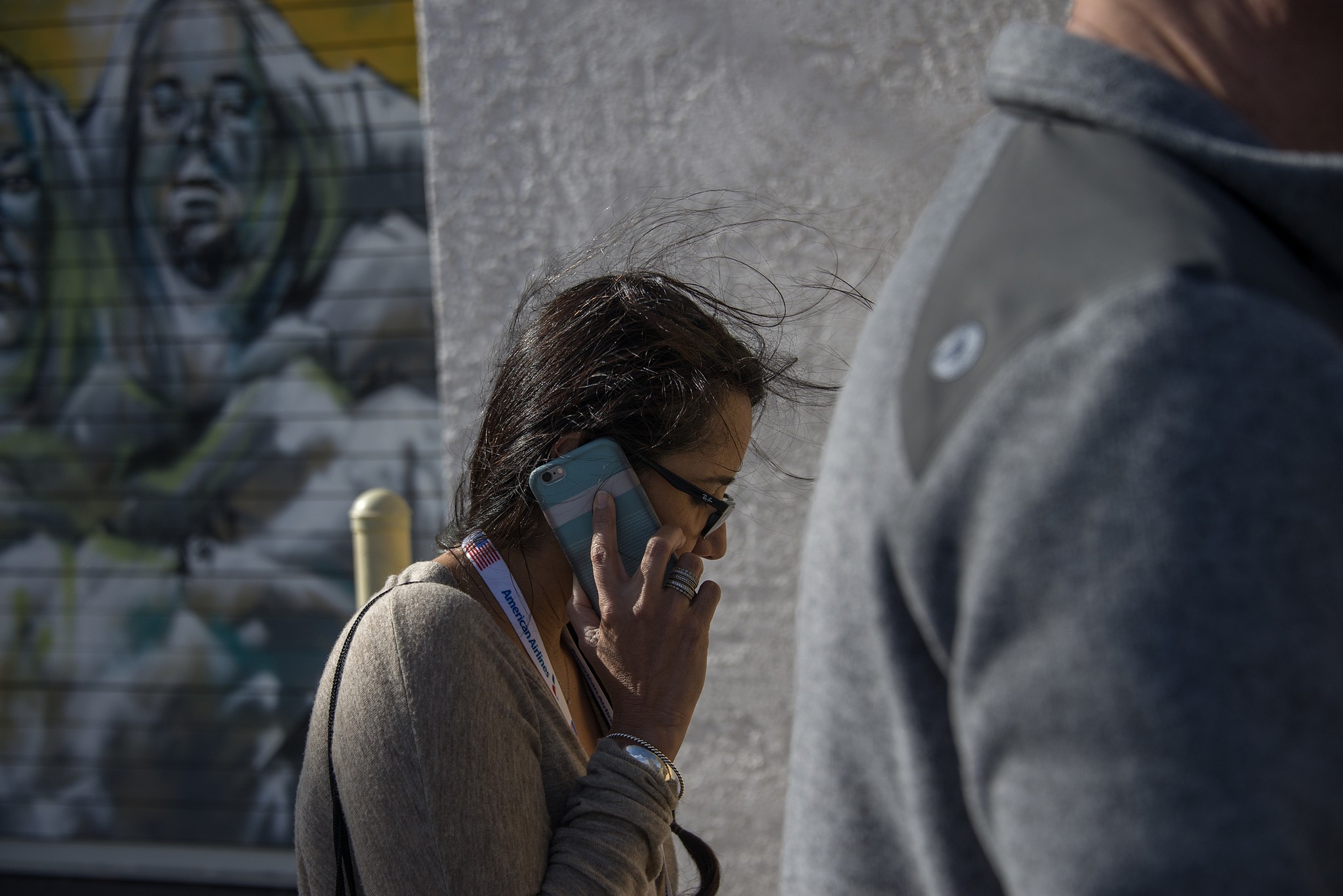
[664,580,694,600]
[669,567,700,592]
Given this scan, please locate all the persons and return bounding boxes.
[290,187,839,896]
[777,0,1343,896]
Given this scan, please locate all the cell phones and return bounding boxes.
[527,437,679,621]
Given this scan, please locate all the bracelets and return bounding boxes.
[603,732,684,800]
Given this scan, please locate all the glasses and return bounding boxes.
[616,438,736,540]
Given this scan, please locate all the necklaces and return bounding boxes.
[556,645,572,704]
